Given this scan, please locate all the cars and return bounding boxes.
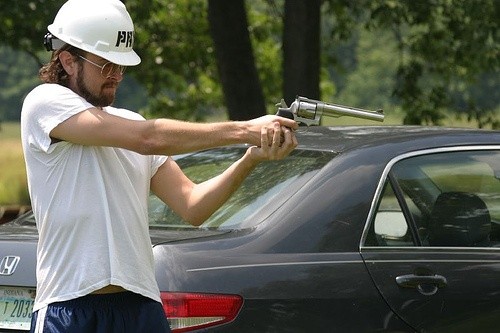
[0,125,500,333]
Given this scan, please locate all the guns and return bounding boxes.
[275,96,386,136]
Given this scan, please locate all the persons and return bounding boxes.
[21,0,298,333]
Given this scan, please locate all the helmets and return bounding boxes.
[46,0,142,66]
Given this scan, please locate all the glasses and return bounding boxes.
[78,54,126,78]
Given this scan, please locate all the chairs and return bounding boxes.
[426,188,494,246]
